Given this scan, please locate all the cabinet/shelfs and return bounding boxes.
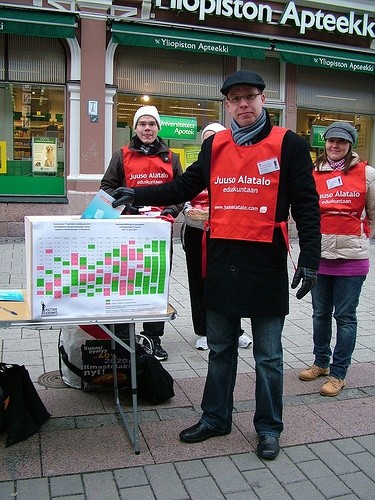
[14,120,46,160]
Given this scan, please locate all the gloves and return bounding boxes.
[291,267,317,300]
[109,187,135,208]
[160,208,175,218]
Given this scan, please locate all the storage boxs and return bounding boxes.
[23,214,172,319]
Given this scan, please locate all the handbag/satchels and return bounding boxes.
[58,324,175,405]
[0,362,51,448]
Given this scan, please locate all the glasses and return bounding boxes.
[226,92,262,103]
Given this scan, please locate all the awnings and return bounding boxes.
[274,40,375,74]
[0,7,76,38]
[111,22,270,60]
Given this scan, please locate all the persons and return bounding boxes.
[180,122,251,350]
[299,121,375,396]
[99,105,185,361]
[111,71,321,459]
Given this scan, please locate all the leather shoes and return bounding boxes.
[179,421,230,443]
[258,435,280,460]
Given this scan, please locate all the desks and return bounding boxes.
[0,287,177,455]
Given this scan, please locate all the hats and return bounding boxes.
[133,106,161,130]
[202,123,226,143]
[220,71,266,97]
[323,122,357,145]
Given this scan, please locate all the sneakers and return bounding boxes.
[320,376,346,396]
[195,335,209,350]
[238,334,252,348]
[143,341,168,360]
[299,363,330,381]
